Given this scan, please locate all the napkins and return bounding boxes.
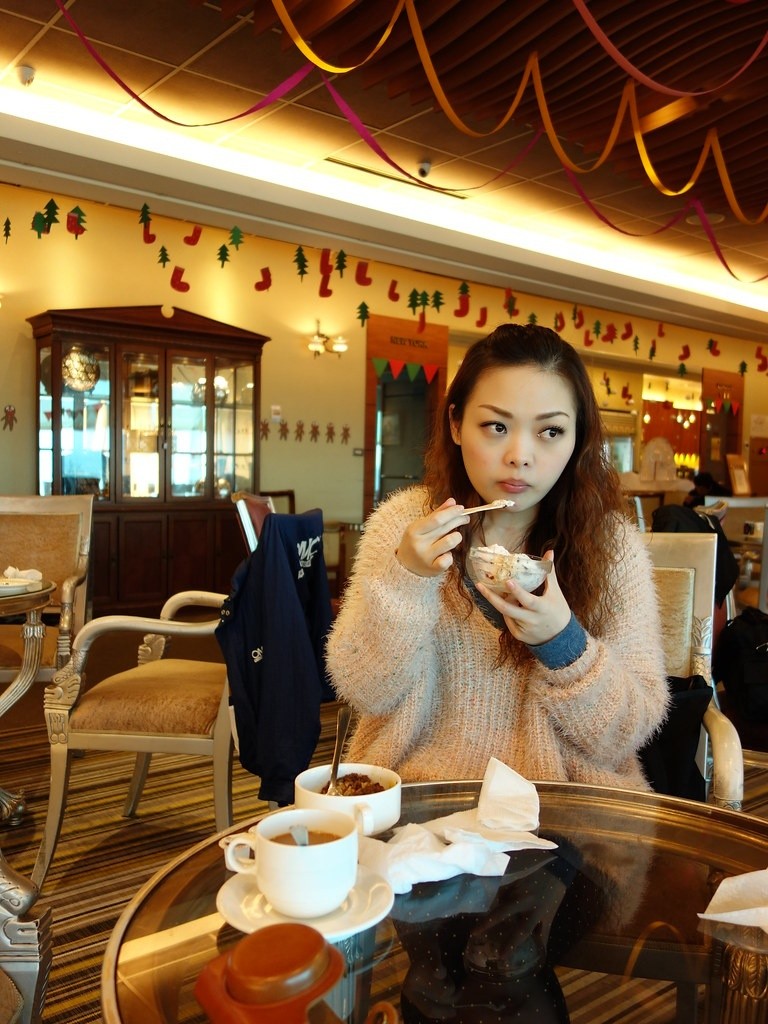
[698,868,768,933]
[3,565,42,580]
[374,757,559,895]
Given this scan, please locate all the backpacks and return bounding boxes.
[653,506,740,607]
[719,608,768,727]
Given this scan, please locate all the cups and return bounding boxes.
[226,807,359,920]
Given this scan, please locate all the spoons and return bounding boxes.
[460,500,515,517]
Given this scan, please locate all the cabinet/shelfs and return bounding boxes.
[25,302,272,621]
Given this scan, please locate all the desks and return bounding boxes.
[0,580,58,828]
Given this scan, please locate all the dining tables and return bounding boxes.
[101,778,768,1024]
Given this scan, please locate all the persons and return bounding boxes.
[395,785,660,1024]
[326,323,669,796]
[683,473,733,508]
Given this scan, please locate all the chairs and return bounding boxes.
[0,490,768,1024]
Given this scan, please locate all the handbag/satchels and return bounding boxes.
[645,673,711,801]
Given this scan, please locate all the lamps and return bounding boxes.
[308,331,348,359]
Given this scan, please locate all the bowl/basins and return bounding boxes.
[294,762,402,837]
[465,546,553,601]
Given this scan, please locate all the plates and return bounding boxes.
[215,861,396,944]
[0,578,37,597]
